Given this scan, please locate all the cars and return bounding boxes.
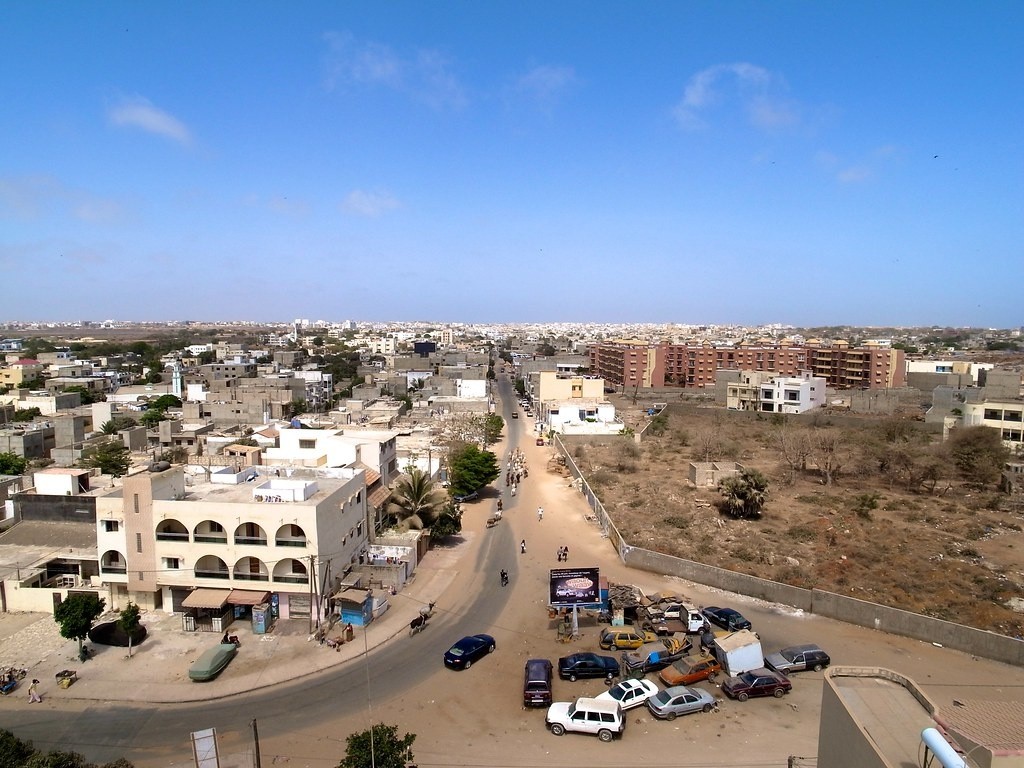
[647,604,683,620]
[646,590,690,608]
[659,652,721,688]
[443,634,497,671]
[536,439,545,446]
[647,686,715,721]
[557,652,620,681]
[524,659,554,709]
[621,638,693,675]
[500,367,533,418]
[594,678,660,711]
[721,667,794,702]
[763,643,831,676]
[512,412,519,419]
[703,605,754,634]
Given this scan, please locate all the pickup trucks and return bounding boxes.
[651,603,712,637]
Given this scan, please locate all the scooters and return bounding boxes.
[221,630,240,647]
[501,570,509,587]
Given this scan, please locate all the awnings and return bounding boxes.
[227,589,269,604]
[181,588,233,609]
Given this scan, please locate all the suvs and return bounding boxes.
[600,626,658,650]
[545,696,627,742]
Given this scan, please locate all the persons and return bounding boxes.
[253,472,258,477]
[500,569,508,586]
[521,540,525,553]
[497,499,503,511]
[506,447,529,497]
[28,679,42,703]
[537,506,543,519]
[342,622,353,642]
[680,392,683,400]
[557,546,569,562]
